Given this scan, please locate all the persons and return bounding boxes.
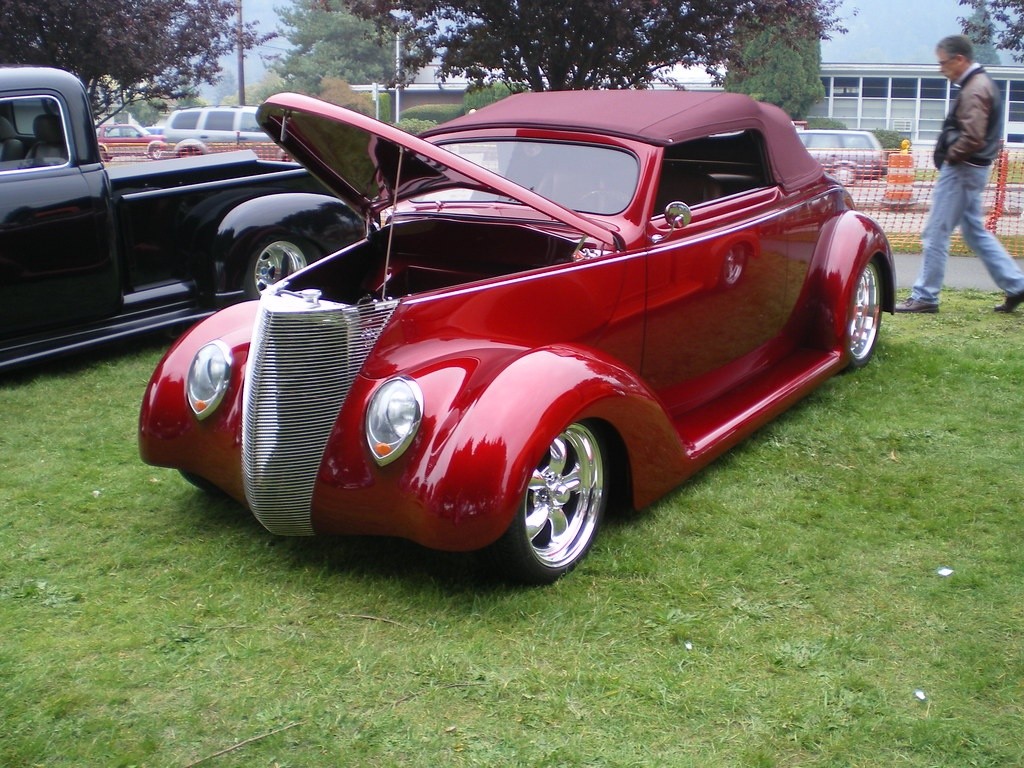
[895,33,1024,314]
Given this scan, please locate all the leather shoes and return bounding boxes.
[894,297,939,313]
[994,290,1023,313]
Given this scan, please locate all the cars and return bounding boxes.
[96,123,167,161]
[137,88,898,585]
[797,130,889,186]
[0,65,367,386]
[164,105,276,144]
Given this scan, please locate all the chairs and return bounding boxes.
[24,114,65,163]
[609,163,725,211]
[0,117,26,165]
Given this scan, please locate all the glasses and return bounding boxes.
[939,57,956,64]
